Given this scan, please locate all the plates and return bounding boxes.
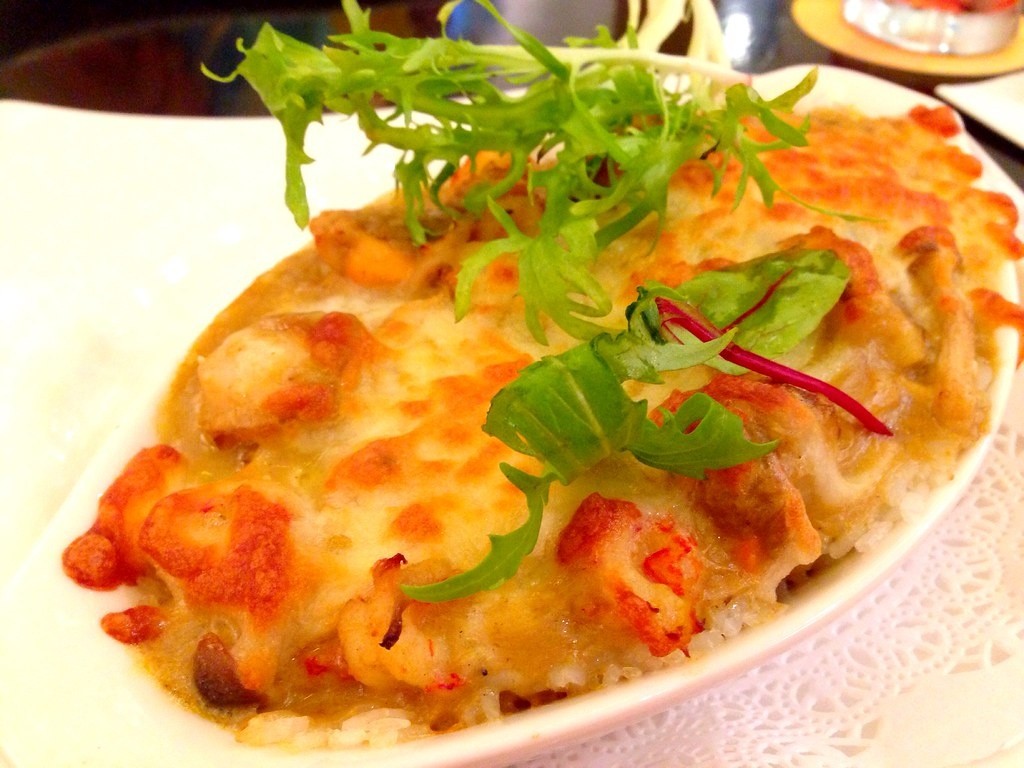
[788,0,1024,78]
[931,66,1021,154]
[0,63,1020,768]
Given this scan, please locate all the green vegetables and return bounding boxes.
[200,1,847,603]
